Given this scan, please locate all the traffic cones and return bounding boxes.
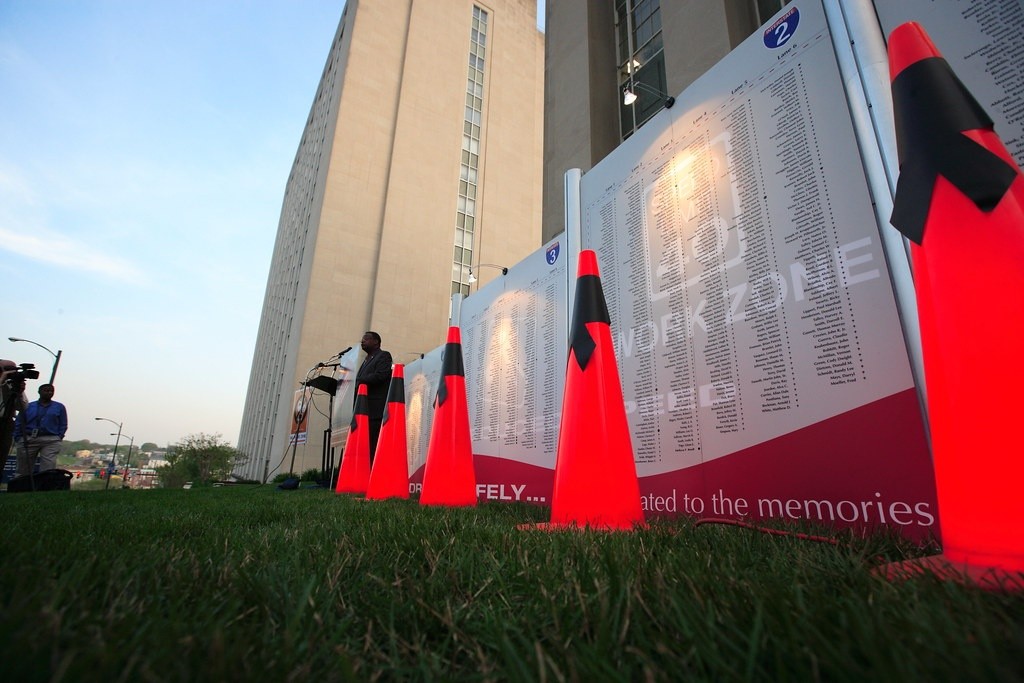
[549,250,652,531]
[366,362,412,501]
[420,326,479,508]
[870,20,1024,593]
[336,382,372,495]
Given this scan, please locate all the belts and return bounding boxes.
[27,432,53,436]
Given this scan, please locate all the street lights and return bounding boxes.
[110,432,134,489]
[8,336,62,397]
[94,417,123,489]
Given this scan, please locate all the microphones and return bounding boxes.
[339,347,353,355]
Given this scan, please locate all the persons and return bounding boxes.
[0,359,29,481]
[354,331,392,469]
[95,468,108,479]
[12,384,68,476]
[128,472,131,480]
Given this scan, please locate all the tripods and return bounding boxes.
[0,391,35,492]
